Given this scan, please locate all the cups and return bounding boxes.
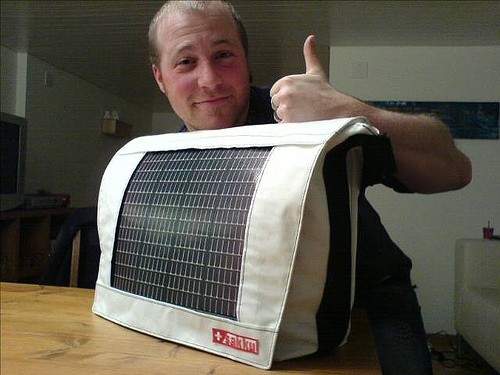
[482,228,494,240]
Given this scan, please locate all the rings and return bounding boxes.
[274,110,282,121]
[271,99,277,110]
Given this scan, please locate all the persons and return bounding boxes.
[147,0,473,375]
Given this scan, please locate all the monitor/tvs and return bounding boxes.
[0,111,28,212]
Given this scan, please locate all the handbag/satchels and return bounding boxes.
[92,115,393,370]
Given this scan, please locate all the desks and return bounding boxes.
[0,208,75,283]
[0,282,382,375]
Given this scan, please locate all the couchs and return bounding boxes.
[454,238,500,375]
